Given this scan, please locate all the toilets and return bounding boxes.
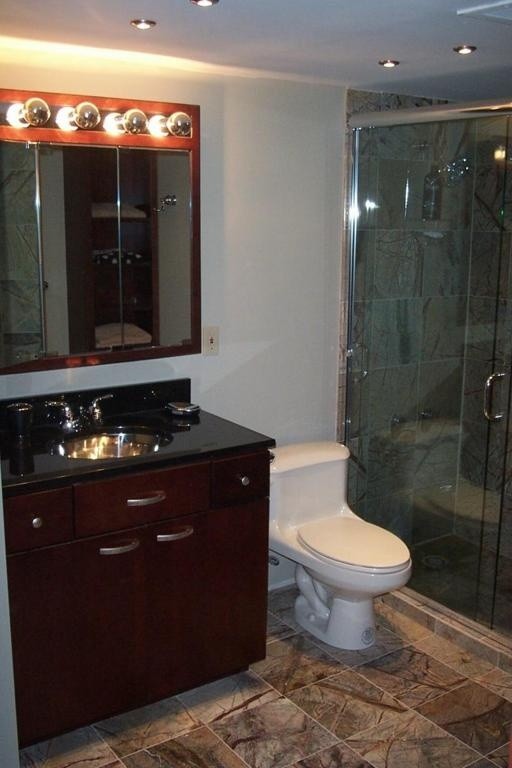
[267,441,413,651]
[380,418,512,533]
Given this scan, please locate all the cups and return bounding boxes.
[6,402,36,436]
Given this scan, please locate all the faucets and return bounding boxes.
[74,407,92,431]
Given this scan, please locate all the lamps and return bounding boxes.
[7,97,193,140]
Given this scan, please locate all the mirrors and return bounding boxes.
[0,136,201,372]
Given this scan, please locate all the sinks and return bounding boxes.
[48,424,174,461]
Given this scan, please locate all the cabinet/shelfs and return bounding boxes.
[59,143,163,353]
[3,452,275,750]
